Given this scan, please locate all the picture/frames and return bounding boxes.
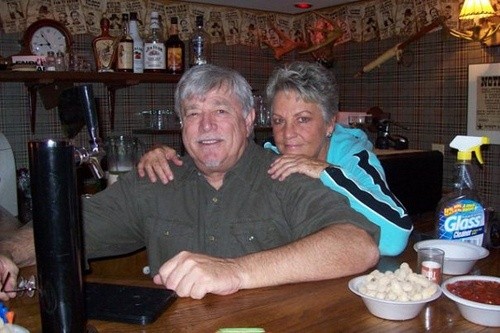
[467,63,500,144]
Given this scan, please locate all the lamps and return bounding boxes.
[458,0,496,27]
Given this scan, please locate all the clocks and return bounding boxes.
[24,19,73,57]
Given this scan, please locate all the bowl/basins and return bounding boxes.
[413,239,489,275]
[441,276,500,326]
[348,275,443,320]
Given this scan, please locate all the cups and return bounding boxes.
[149,94,277,130]
[418,247,444,286]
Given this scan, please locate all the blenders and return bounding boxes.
[102,136,146,188]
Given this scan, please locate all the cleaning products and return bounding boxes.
[434,135,491,248]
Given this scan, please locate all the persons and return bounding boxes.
[0,63,382,302]
[137,61,414,256]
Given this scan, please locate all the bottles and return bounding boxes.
[165,17,185,72]
[118,14,133,72]
[143,11,167,73]
[93,17,119,72]
[46,52,55,71]
[188,16,210,68]
[55,52,65,71]
[129,12,144,72]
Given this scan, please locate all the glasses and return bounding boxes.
[1,272,38,298]
